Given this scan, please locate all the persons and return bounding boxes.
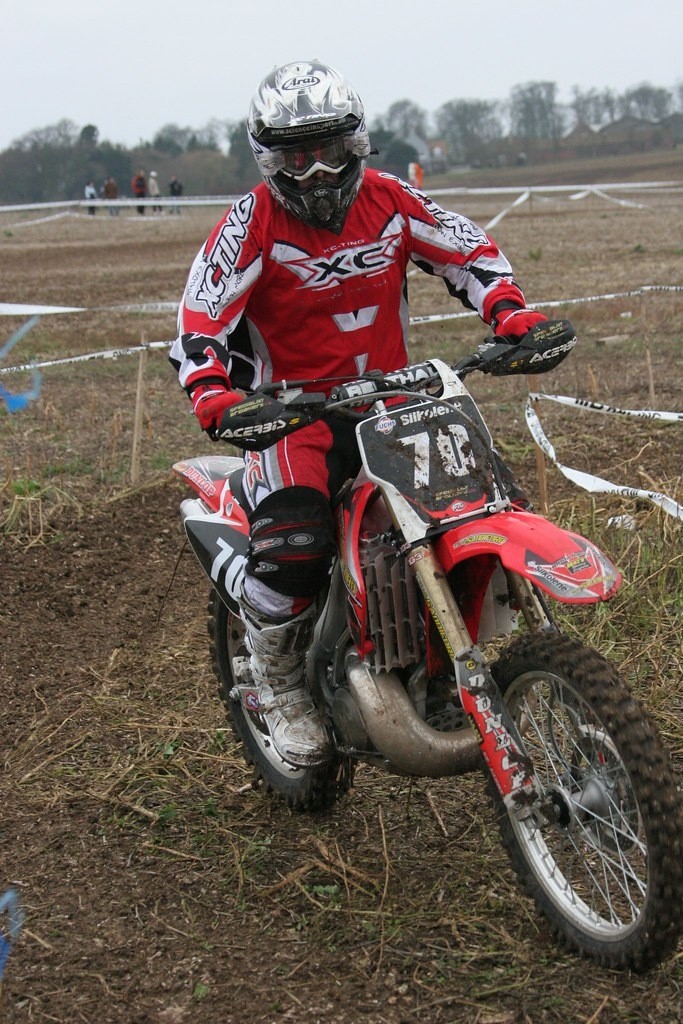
[406,161,423,191]
[168,61,553,765]
[85,170,183,216]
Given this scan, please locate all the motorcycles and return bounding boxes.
[168,319,683,973]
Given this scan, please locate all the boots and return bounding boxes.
[238,585,334,769]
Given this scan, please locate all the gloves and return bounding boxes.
[494,307,548,343]
[189,383,245,429]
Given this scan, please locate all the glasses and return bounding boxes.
[270,136,352,181]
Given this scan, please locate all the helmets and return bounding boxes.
[248,61,371,228]
